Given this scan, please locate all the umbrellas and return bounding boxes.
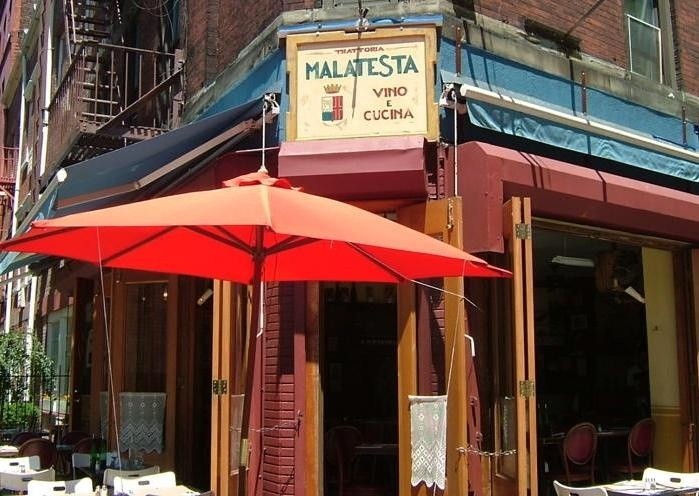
[0,166,514,496]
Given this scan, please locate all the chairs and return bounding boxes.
[0,431,212,495]
[542,422,598,496]
[324,420,400,495]
[607,417,656,481]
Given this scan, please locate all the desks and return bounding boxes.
[539,430,628,496]
